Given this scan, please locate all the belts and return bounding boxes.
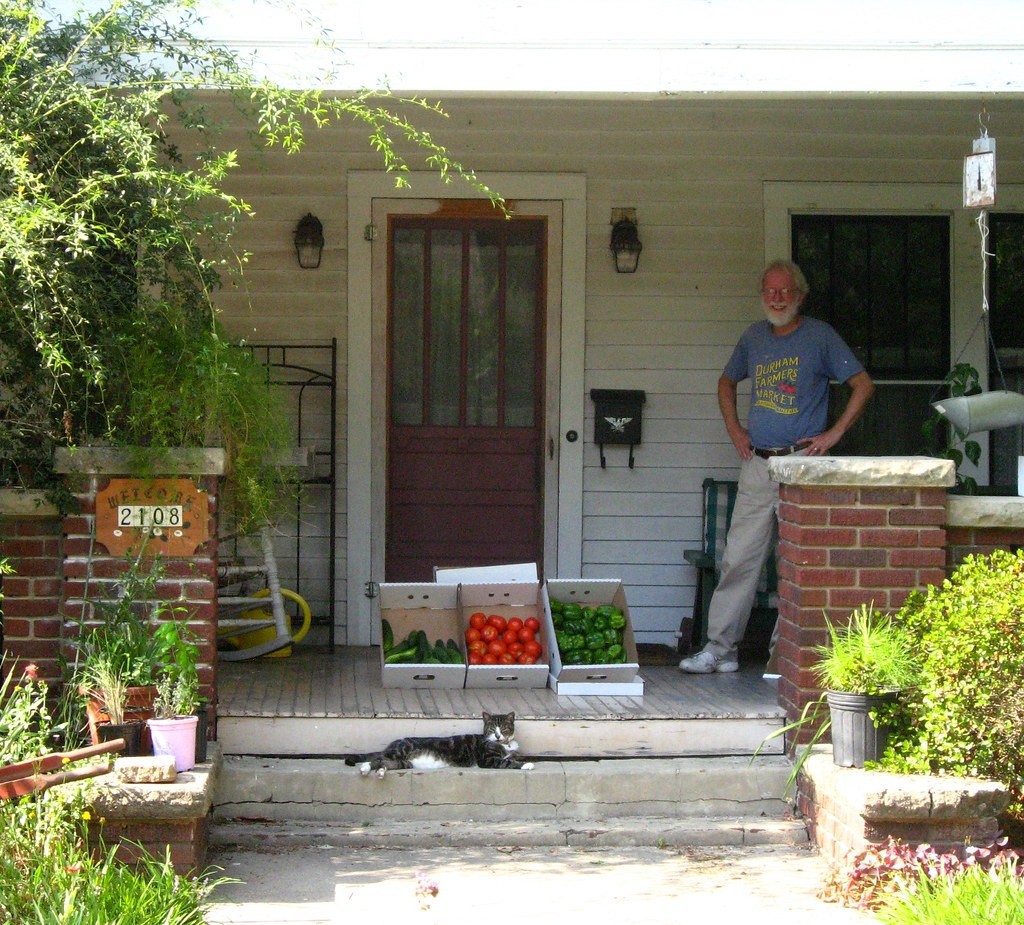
[750,440,813,460]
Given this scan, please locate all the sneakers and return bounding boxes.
[678,650,739,673]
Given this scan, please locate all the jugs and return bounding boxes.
[218,587,311,656]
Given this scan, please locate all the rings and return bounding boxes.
[814,447,818,450]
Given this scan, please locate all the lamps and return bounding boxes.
[609,204,642,274]
[928,310,1024,435]
[294,213,324,270]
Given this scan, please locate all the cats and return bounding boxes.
[344,711,536,780]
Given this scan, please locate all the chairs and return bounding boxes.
[683,476,778,671]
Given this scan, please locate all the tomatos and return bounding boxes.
[466,613,542,665]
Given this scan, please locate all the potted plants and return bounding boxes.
[61,546,210,772]
[810,598,924,771]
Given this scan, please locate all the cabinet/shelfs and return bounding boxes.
[226,338,337,653]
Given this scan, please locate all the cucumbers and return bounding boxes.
[382,619,464,664]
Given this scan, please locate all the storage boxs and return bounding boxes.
[548,671,645,697]
[432,563,539,585]
[377,582,468,690]
[459,581,550,688]
[542,578,640,683]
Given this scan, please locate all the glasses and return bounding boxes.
[759,288,801,296]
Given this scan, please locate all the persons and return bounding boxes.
[679,256,876,673]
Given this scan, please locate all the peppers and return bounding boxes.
[549,598,625,664]
